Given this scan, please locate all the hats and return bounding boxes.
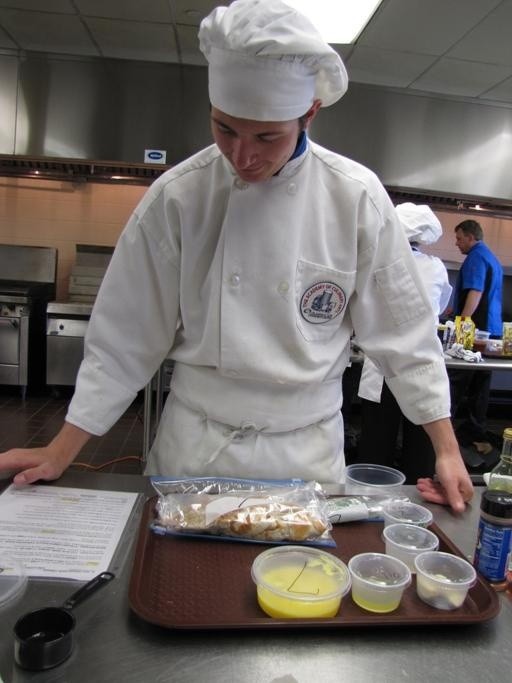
[395,202,442,246]
[198,0,348,122]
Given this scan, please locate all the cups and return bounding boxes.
[502,321,512,353]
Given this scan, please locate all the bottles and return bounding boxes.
[471,428,511,591]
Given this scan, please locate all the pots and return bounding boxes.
[13,569,115,668]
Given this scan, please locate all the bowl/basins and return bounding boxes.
[252,460,478,618]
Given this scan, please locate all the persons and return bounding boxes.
[1,0,476,518]
[438,215,506,338]
[352,198,455,476]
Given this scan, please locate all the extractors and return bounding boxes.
[1,155,511,216]
